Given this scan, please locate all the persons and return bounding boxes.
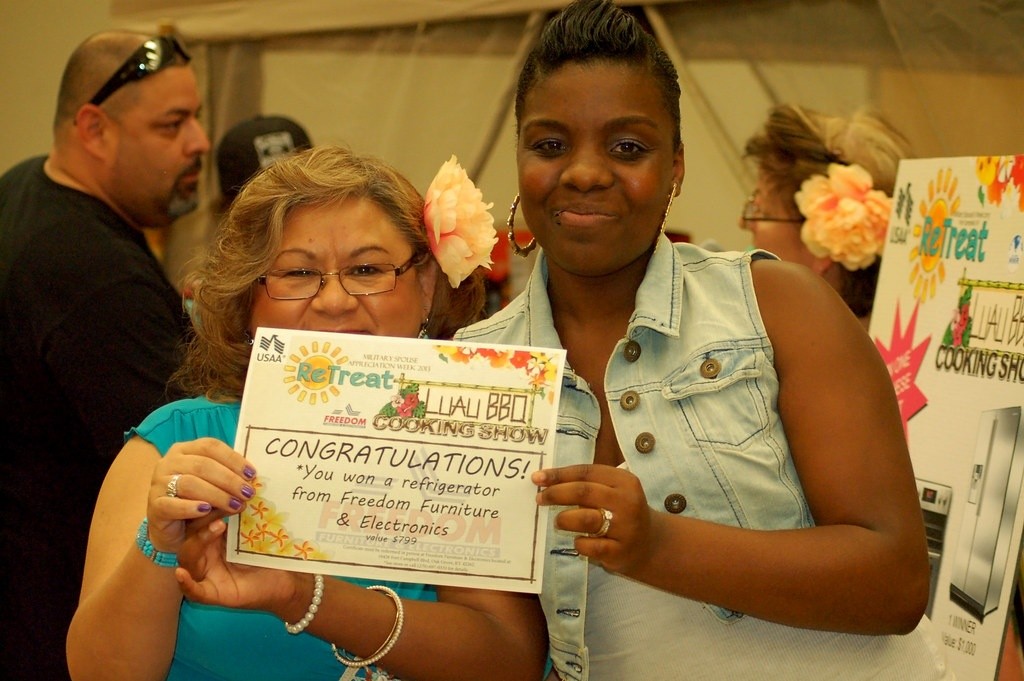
[739,100,912,330]
[210,114,313,213]
[0,26,199,681]
[66,142,554,681]
[173,0,955,681]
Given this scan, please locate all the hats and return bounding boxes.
[216,117,313,199]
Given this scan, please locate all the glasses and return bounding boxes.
[257,248,421,301]
[73,34,192,127]
[739,188,806,231]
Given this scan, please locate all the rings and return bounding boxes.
[168,473,181,497]
[588,509,613,538]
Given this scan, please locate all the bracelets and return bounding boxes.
[285,573,324,633]
[136,519,180,568]
[332,585,404,669]
